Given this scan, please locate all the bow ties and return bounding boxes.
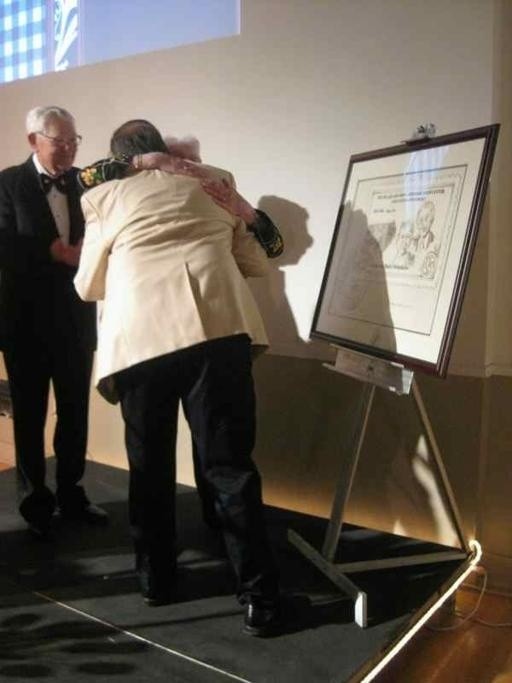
[38,172,67,195]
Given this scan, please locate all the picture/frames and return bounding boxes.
[307,123,507,380]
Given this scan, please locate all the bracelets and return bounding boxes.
[137,154,142,169]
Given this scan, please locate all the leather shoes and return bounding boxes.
[243,595,311,638]
[29,514,56,541]
[140,581,167,604]
[62,504,109,524]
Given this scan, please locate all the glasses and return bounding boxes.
[36,130,82,148]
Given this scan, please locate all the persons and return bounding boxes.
[393,196,440,271]
[76,135,285,524]
[70,119,314,637]
[0,104,109,538]
